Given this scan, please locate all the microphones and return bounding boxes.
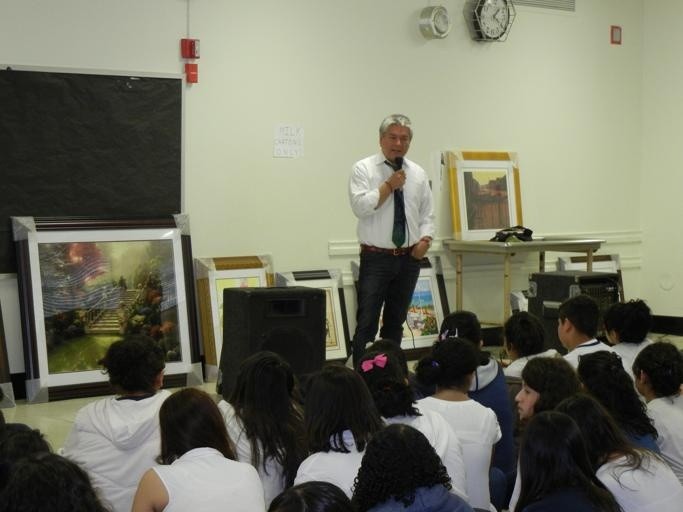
[395,156,403,200]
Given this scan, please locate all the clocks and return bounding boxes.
[469,0,518,41]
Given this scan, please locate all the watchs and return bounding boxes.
[419,238,433,249]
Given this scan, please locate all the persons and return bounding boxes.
[369,338,425,402]
[0,414,53,488]
[353,350,465,504]
[440,311,514,510]
[556,294,630,387]
[496,311,564,406]
[632,341,681,484]
[507,356,584,512]
[513,412,625,512]
[413,336,507,511]
[130,388,266,511]
[216,352,304,510]
[292,366,387,501]
[348,114,437,370]
[52,333,176,512]
[352,422,475,510]
[269,481,352,512]
[1,449,103,510]
[577,352,663,458]
[603,299,665,404]
[550,394,682,512]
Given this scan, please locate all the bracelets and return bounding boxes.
[384,180,393,193]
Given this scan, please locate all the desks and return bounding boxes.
[440,235,604,352]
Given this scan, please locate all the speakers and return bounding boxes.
[527,270,619,355]
[222,286,326,403]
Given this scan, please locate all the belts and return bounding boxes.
[361,243,413,256]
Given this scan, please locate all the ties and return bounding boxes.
[384,160,405,248]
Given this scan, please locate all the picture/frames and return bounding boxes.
[8,214,204,405]
[445,150,524,240]
[336,256,457,363]
[557,254,625,304]
[192,256,273,385]
[273,270,351,368]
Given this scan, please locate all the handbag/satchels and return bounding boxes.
[497,226,532,241]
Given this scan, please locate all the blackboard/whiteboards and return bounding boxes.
[0,64,186,275]
